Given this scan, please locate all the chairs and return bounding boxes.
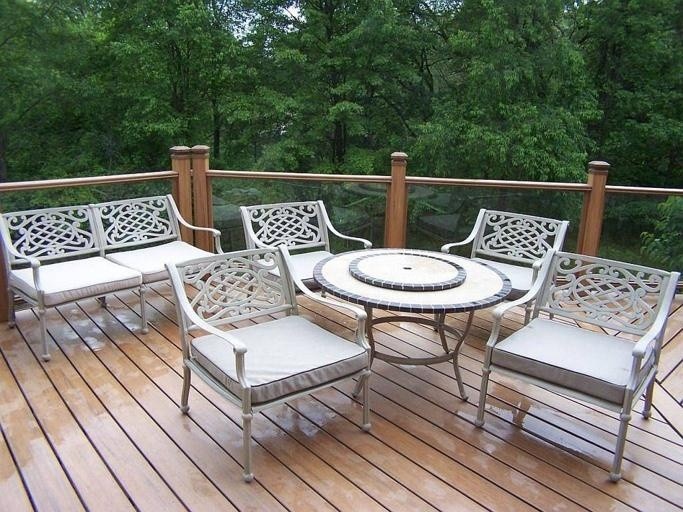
[238,199,373,316]
[473,249,680,482]
[164,245,372,481]
[88,193,227,334]
[0,204,149,361]
[211,180,521,252]
[430,208,570,332]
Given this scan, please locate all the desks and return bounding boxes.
[312,248,512,401]
